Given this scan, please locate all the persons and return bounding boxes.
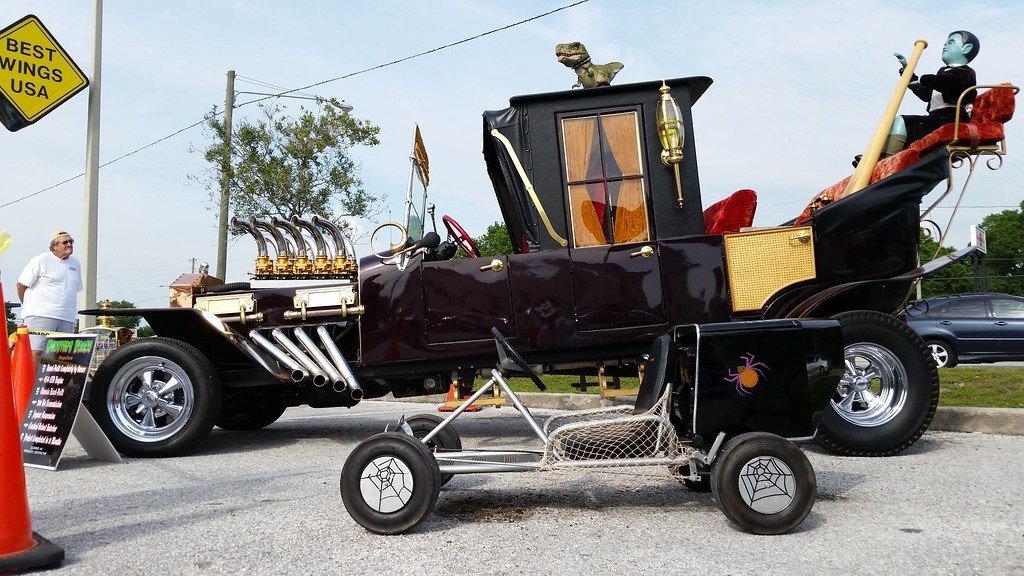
[852,29,982,171]
[16,229,85,372]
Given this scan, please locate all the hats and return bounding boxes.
[49,230,69,244]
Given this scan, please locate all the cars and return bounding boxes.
[896,291,1024,369]
[74,28,1020,537]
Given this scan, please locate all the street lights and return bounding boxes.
[216,69,353,283]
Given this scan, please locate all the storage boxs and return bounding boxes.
[721,224,819,316]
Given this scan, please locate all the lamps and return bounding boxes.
[655,77,686,210]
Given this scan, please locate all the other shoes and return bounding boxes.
[852,160,858,168]
[854,155,862,162]
